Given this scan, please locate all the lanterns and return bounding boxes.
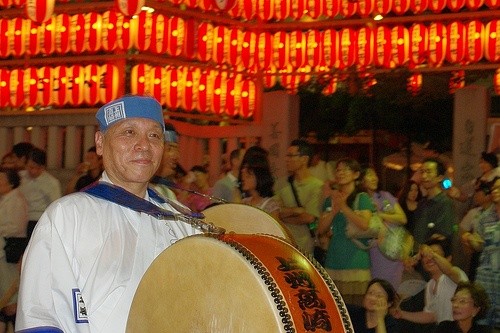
[0,0,500,119]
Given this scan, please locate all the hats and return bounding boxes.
[95,95,168,133]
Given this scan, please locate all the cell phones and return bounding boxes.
[441,178,452,191]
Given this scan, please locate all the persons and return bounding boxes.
[0,165,40,333]
[0,139,61,239]
[67,144,102,195]
[173,139,500,333]
[15,96,209,333]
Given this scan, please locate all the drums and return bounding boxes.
[195,202,298,247]
[125,232,354,333]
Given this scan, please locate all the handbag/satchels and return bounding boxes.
[4,237,28,263]
[314,246,327,261]
[345,192,383,249]
[379,220,414,261]
